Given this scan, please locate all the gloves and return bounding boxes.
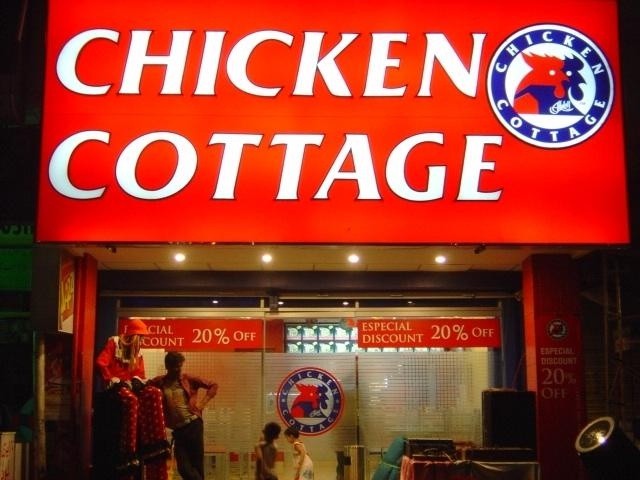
[132,378,143,392]
[113,380,127,387]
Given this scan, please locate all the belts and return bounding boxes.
[175,414,197,427]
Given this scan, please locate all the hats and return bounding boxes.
[124,320,149,338]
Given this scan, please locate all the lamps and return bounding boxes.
[574,416,640,480]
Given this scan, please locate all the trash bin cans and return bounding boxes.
[342,445,363,479]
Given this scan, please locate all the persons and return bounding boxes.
[145,350,220,479]
[284,424,315,480]
[95,318,171,471]
[254,421,281,480]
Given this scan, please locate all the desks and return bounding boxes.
[400,455,538,480]
